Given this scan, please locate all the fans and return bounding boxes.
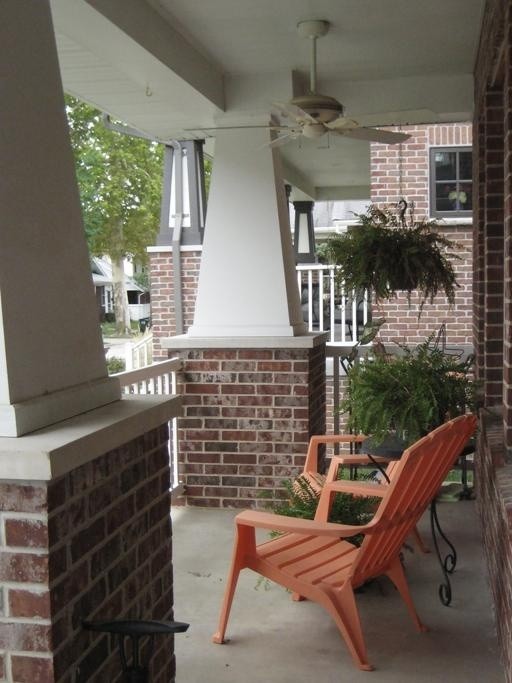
[181,19,412,148]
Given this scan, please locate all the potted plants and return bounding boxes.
[314,202,474,322]
[258,464,415,594]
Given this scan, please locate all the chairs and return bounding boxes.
[212,415,476,672]
[294,413,451,591]
